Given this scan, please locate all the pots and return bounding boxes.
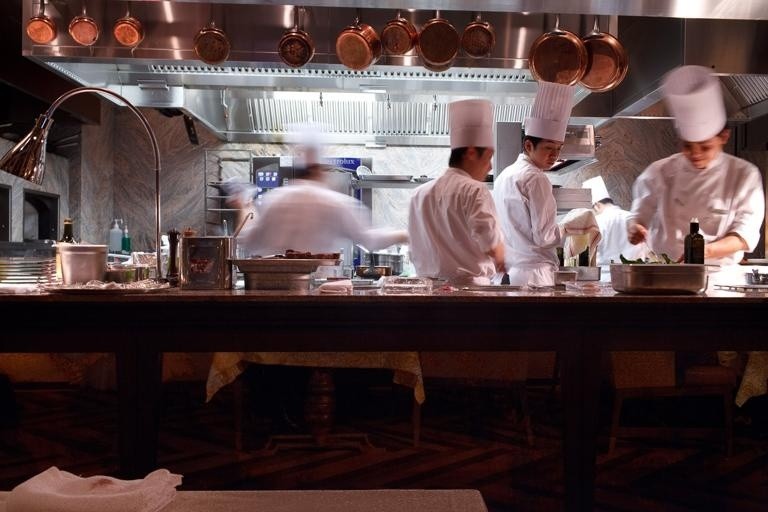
[461,13,496,63]
[381,9,417,57]
[575,15,629,94]
[528,14,588,88]
[26,1,57,45]
[335,9,383,71]
[277,6,316,69]
[194,3,231,66]
[113,0,142,48]
[69,1,102,47]
[416,10,460,73]
[371,253,403,276]
[356,266,393,280]
[559,266,601,281]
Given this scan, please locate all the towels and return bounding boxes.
[5,465,184,511]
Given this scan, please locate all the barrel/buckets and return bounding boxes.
[59,244,109,285]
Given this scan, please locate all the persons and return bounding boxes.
[581,156,636,267]
[492,82,593,287]
[408,100,507,285]
[237,122,407,281]
[625,64,765,273]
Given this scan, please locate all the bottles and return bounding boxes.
[684,219,704,263]
[110,217,123,252]
[121,224,130,256]
[60,218,76,244]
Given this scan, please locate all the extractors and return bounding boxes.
[610,17,768,125]
[21,45,592,148]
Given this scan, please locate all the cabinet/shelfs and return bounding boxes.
[204,147,255,231]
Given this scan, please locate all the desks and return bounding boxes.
[0,488,489,511]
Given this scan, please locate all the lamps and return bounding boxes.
[0,87,163,279]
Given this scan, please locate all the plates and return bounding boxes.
[414,178,434,182]
[383,284,431,290]
[0,258,55,291]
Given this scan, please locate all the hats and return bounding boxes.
[285,121,329,165]
[582,176,611,206]
[525,82,575,142]
[448,99,497,152]
[664,65,727,140]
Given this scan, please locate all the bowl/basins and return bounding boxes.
[744,272,768,284]
